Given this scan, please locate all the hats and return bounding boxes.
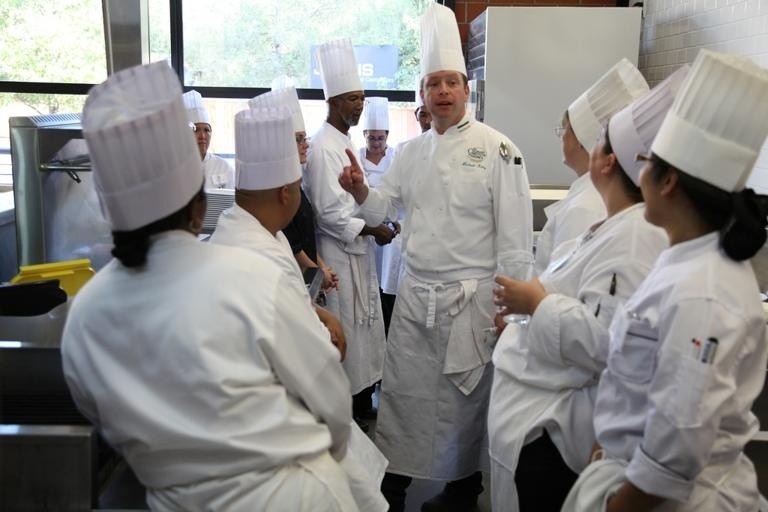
[607,63,691,188]
[81,60,204,233]
[414,74,424,113]
[234,107,302,191]
[651,48,768,194]
[319,37,364,102]
[567,58,650,155]
[419,2,468,83]
[183,90,212,131]
[248,86,307,136]
[363,96,389,132]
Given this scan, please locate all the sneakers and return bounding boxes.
[354,416,369,432]
[362,407,377,419]
[421,491,478,512]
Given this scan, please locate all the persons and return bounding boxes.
[337,0,534,512]
[487,47,767,512]
[60,38,432,512]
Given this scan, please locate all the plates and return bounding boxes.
[202,188,236,230]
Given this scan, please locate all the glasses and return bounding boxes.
[554,124,569,136]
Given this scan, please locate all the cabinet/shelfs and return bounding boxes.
[465,7,642,188]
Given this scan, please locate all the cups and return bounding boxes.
[495,250,536,324]
